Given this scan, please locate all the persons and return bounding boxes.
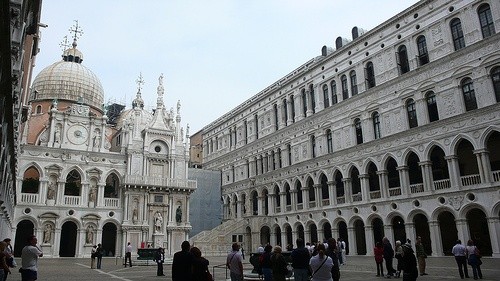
[259,243,289,281]
[91,244,104,269]
[0,238,13,281]
[227,243,244,281]
[48,182,55,199]
[290,237,346,281]
[156,247,165,276]
[86,225,93,243]
[94,132,100,147]
[452,239,482,279]
[172,241,209,281]
[44,225,51,243]
[125,242,132,267]
[55,127,60,143]
[177,206,182,222]
[156,213,162,230]
[374,236,428,281]
[90,185,96,205]
[21,236,43,281]
[133,206,137,219]
